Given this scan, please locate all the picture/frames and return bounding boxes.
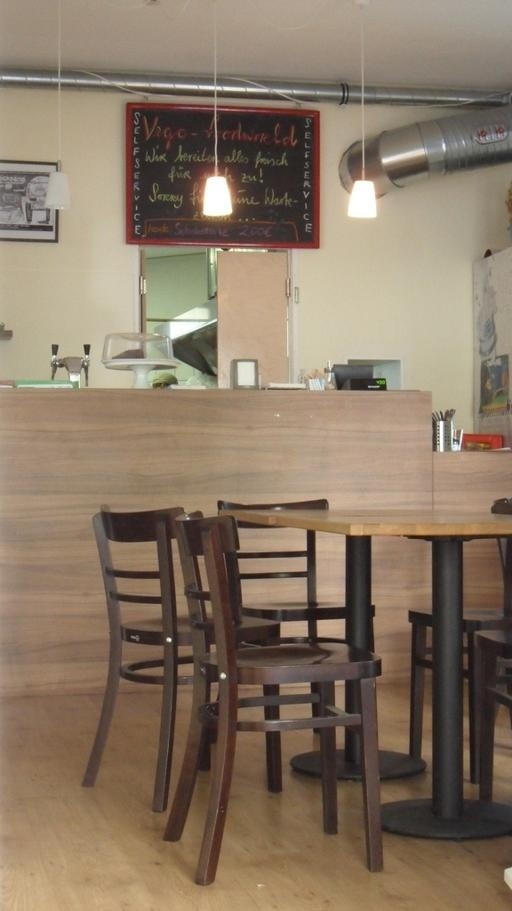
[0,158,60,245]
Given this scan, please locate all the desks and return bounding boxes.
[222,499,508,845]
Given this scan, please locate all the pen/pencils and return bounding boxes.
[432,409,456,421]
[305,373,320,379]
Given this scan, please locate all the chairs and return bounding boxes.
[79,502,286,812]
[470,629,511,807]
[162,509,391,888]
[217,492,331,790]
[406,497,512,762]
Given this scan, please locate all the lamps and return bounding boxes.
[196,1,238,217]
[346,2,380,218]
[43,0,71,213]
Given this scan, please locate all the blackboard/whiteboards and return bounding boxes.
[126,102,321,248]
